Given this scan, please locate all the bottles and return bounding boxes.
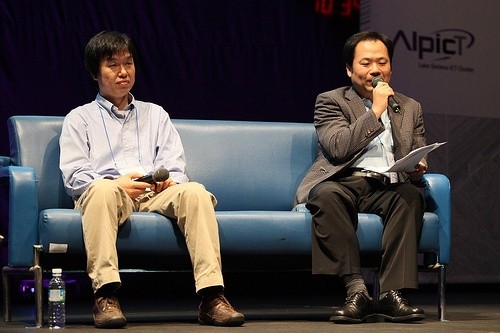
[47,268,66,329]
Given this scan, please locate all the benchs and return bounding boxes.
[0,116,452,327]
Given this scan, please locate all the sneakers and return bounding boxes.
[198,292,245,326]
[92,296,127,328]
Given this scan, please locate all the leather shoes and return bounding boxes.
[376,289,426,322]
[329,292,378,323]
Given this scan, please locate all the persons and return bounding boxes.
[59,28,244,326]
[294,30,428,321]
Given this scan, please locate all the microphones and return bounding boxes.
[133,169,170,184]
[371,77,400,113]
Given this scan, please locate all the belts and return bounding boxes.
[345,167,391,185]
